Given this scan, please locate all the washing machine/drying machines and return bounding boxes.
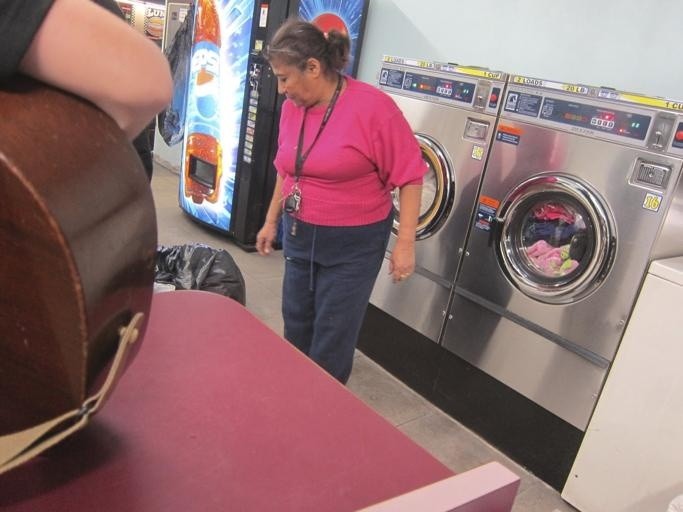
[441,74,682,433]
[368,55,508,344]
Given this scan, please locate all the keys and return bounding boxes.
[278,189,301,213]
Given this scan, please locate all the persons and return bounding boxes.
[406,273,411,276]
[256,19,430,387]
[401,275,406,278]
[1,0,173,183]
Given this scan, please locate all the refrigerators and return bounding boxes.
[178,0,370,255]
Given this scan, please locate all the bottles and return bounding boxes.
[184,0,225,204]
